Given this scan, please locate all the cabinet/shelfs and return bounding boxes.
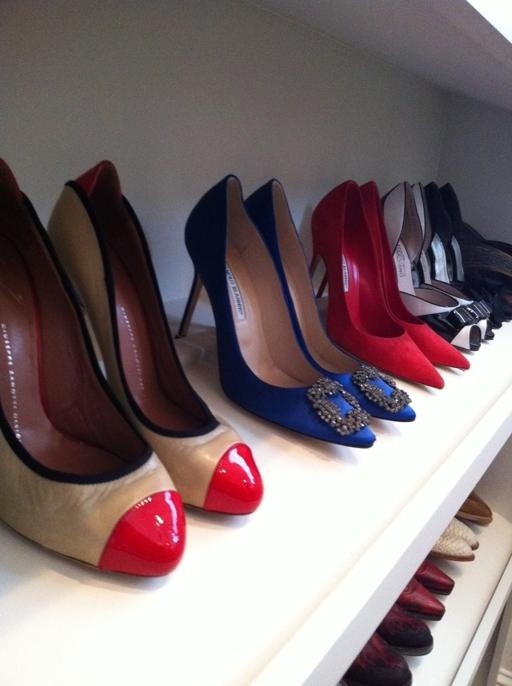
[1,0,512,686]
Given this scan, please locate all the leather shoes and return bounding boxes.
[340,489,492,681]
